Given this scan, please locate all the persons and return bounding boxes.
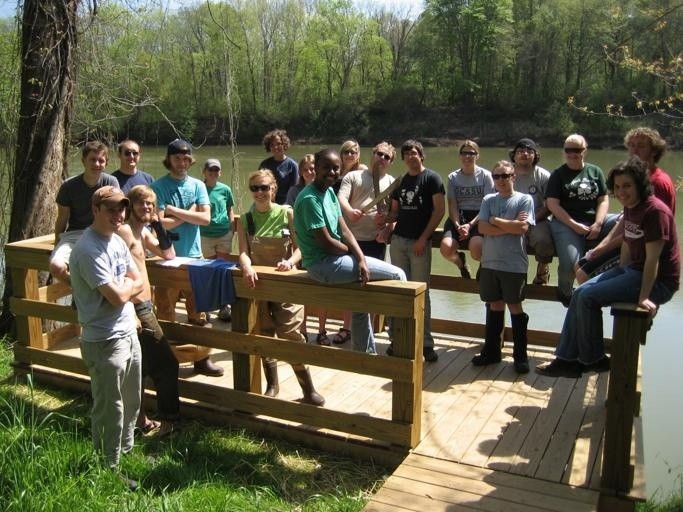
[293,151,408,354]
[508,138,554,284]
[260,129,368,341]
[441,140,495,280]
[573,128,675,283]
[49,141,120,310]
[117,185,182,435]
[535,162,678,376]
[111,141,155,197]
[72,186,154,487]
[238,170,326,406]
[470,162,535,375]
[334,141,395,343]
[151,140,224,376]
[199,158,235,321]
[386,140,445,360]
[544,135,620,306]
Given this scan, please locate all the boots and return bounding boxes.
[472,309,506,367]
[263,361,279,397]
[187,319,224,377]
[510,312,530,374]
[293,365,326,408]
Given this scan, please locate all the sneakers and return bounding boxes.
[534,354,611,379]
[386,339,439,363]
[459,252,471,279]
[101,452,156,490]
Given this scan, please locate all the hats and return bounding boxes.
[92,185,130,209]
[204,158,221,171]
[167,139,193,156]
[513,138,537,152]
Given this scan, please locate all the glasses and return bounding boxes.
[341,147,585,179]
[119,152,139,156]
[249,185,275,193]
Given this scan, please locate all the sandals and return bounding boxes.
[533,262,551,286]
[300,328,351,346]
[137,419,174,438]
